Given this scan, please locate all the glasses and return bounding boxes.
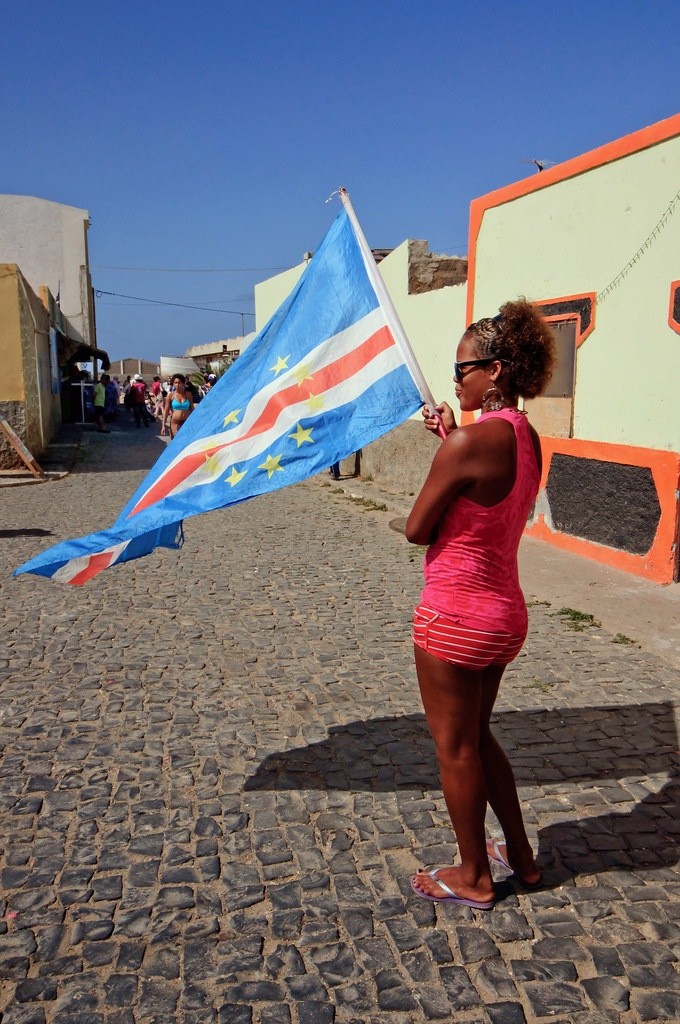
[455,358,510,383]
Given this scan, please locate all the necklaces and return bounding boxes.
[511,409,528,414]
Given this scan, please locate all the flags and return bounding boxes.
[14,206,424,587]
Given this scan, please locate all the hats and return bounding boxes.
[134,374,140,381]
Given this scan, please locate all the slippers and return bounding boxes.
[410,868,494,909]
[486,838,545,890]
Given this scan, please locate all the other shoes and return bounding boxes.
[98,429,110,433]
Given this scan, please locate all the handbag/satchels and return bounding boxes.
[162,390,167,397]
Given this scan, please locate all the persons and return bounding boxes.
[77,370,216,433]
[329,462,340,479]
[161,374,195,440]
[406,296,554,908]
